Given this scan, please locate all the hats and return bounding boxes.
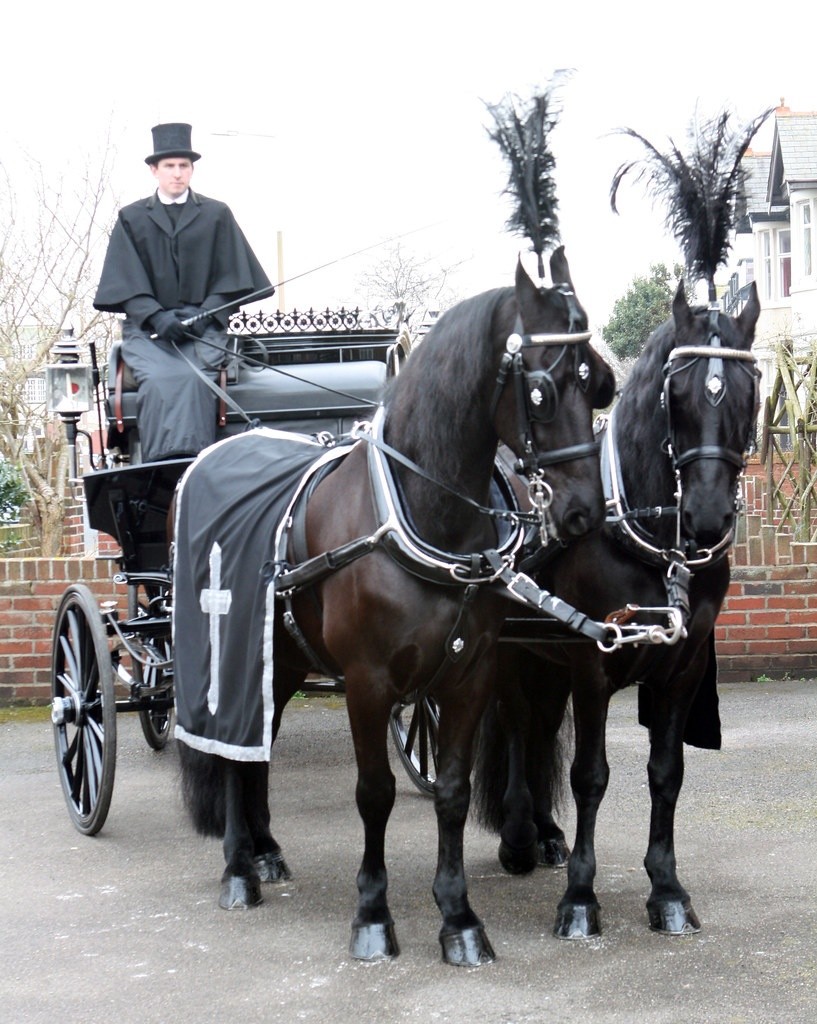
[144,123,202,165]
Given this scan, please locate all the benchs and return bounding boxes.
[103,328,405,425]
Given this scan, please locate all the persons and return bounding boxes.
[93,123,275,464]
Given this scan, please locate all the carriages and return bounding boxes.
[44,70,785,970]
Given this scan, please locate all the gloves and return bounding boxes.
[147,309,193,346]
[176,306,215,340]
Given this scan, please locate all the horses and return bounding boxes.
[168,245,606,969]
[475,278,761,940]
